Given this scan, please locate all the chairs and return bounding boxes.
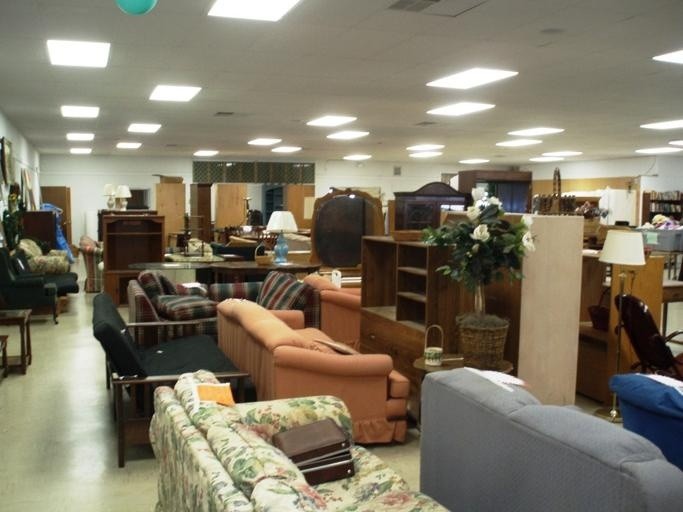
[1,244,82,326]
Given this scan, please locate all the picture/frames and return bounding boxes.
[1,135,21,188]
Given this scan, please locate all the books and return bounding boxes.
[664,255,676,264]
[650,190,683,213]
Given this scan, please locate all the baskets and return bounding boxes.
[424,325,444,366]
[459,319,510,371]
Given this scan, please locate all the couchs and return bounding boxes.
[94,270,683,512]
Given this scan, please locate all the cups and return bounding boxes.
[423,348,443,366]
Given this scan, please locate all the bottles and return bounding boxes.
[274,230,288,263]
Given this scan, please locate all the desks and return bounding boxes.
[1,308,34,376]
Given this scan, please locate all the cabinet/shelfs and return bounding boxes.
[358,166,683,403]
[18,174,330,305]
